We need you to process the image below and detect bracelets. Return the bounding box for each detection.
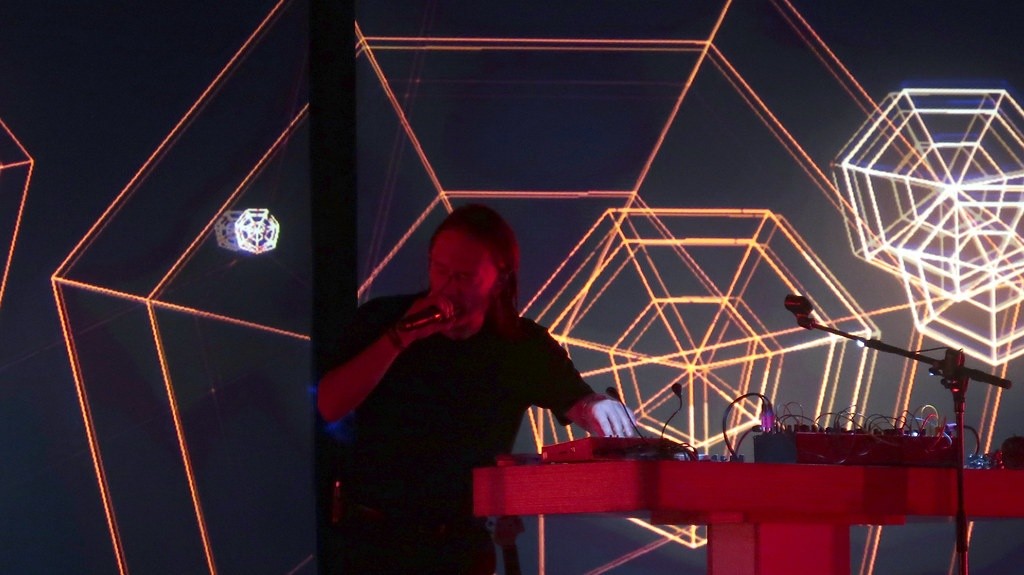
[387,326,407,354]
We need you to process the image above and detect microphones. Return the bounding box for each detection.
[660,383,683,439]
[606,387,644,439]
[397,306,442,333]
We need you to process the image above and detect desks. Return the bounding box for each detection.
[473,460,1024,575]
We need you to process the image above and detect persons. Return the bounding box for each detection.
[314,204,635,575]
